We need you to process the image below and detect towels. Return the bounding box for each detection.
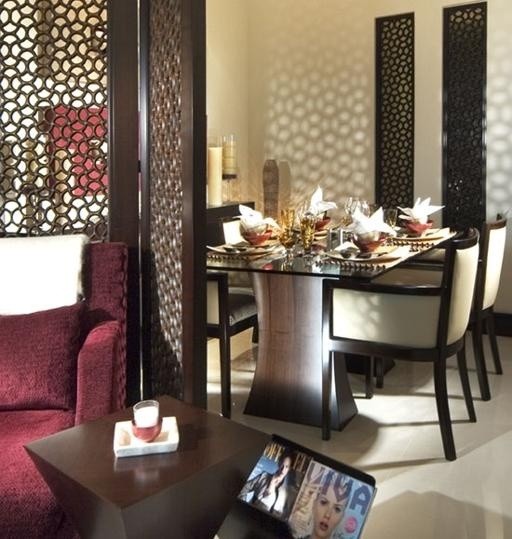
[308,185,338,219]
[336,205,399,237]
[232,203,282,232]
[396,196,446,225]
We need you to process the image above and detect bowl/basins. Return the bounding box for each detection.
[241,230,272,245]
[351,236,386,251]
[401,219,433,238]
[316,217,331,230]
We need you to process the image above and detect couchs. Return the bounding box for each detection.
[0,234,129,539]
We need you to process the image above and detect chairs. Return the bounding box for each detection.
[322,228,480,460]
[205,215,259,418]
[374,212,508,401]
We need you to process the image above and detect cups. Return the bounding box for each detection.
[132,416,162,443]
[133,399,159,427]
[344,196,397,229]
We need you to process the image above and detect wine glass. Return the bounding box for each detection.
[278,232,299,270]
[300,219,316,258]
[279,208,296,235]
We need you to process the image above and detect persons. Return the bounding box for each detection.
[237,448,298,517]
[299,469,354,538]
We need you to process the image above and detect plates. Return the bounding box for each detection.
[325,244,411,261]
[205,239,279,253]
[392,225,451,241]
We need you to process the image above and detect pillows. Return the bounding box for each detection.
[0,299,84,411]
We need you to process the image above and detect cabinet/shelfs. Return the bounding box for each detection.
[206,200,257,255]
